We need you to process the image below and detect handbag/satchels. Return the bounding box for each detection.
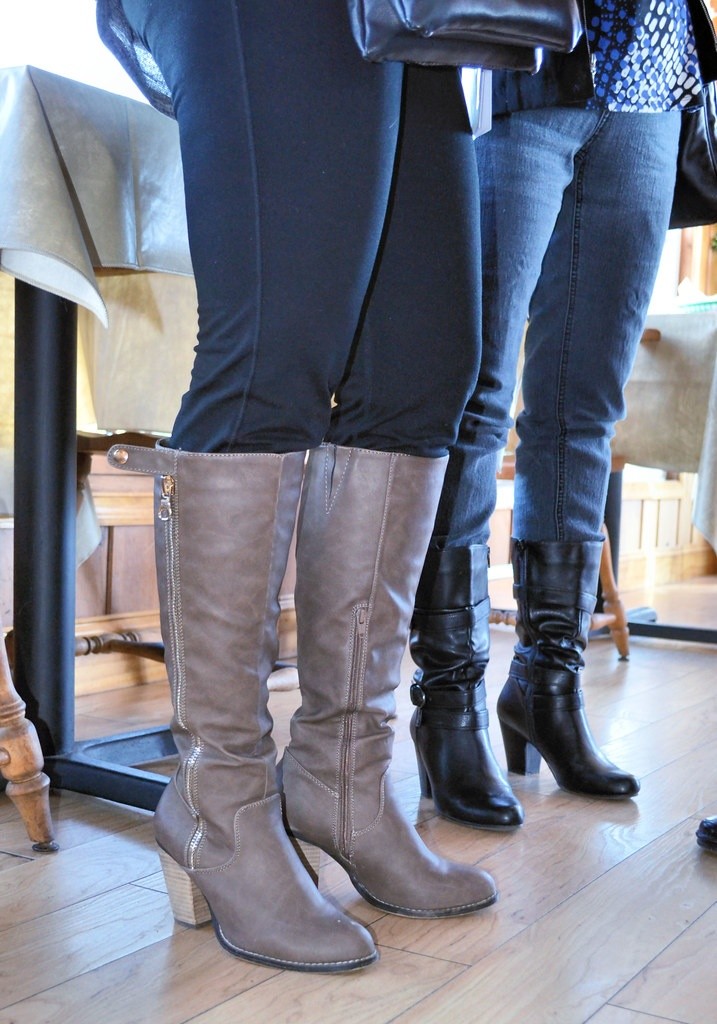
[348,0,584,74]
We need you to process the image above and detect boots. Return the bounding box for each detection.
[108,439,378,970]
[420,545,524,831]
[498,537,639,800]
[279,443,499,919]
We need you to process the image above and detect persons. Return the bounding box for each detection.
[96,0,500,973]
[408,0,717,833]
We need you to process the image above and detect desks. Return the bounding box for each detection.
[0,63,198,812]
[490,309,716,645]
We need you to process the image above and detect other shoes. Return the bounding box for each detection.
[696,815,717,852]
